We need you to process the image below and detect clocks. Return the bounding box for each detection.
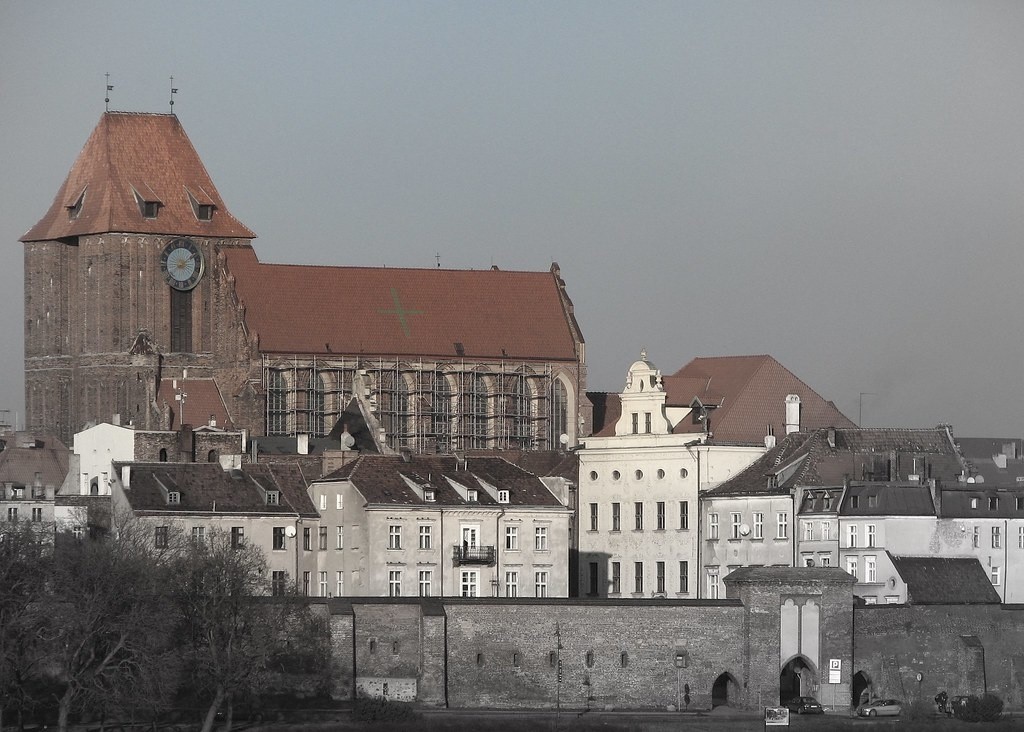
[158,236,205,292]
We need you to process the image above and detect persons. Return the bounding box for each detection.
[946,700,965,720]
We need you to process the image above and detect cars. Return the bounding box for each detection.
[785,696,822,714]
[856,698,906,718]
